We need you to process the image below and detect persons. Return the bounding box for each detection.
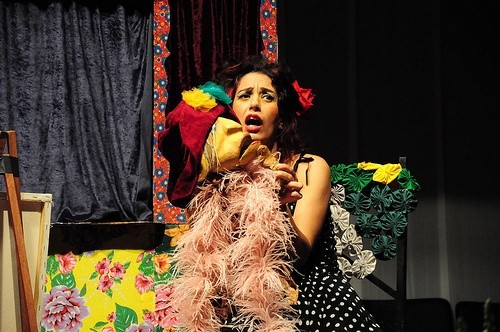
[228,58,385,332]
